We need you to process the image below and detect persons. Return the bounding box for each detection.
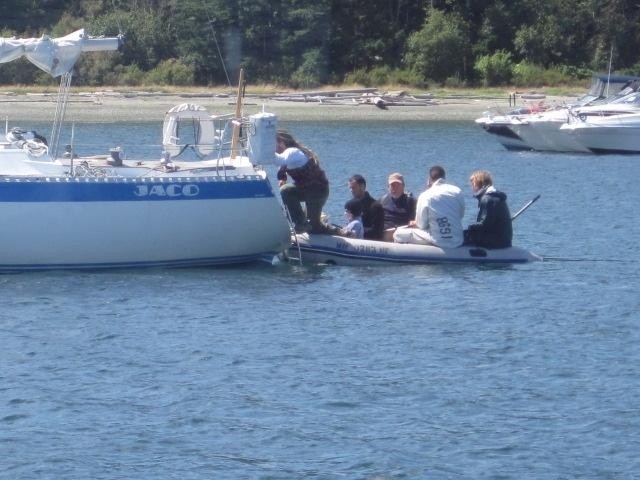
[376,170,418,241]
[273,128,330,234]
[348,174,385,241]
[391,165,466,250]
[464,169,512,248]
[338,198,366,240]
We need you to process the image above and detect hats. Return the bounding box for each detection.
[388,172,404,184]
[344,199,361,217]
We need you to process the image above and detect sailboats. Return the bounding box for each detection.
[1,29,291,274]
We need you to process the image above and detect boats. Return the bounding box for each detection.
[476,110,529,153]
[512,107,593,155]
[562,93,639,152]
[293,233,542,266]
